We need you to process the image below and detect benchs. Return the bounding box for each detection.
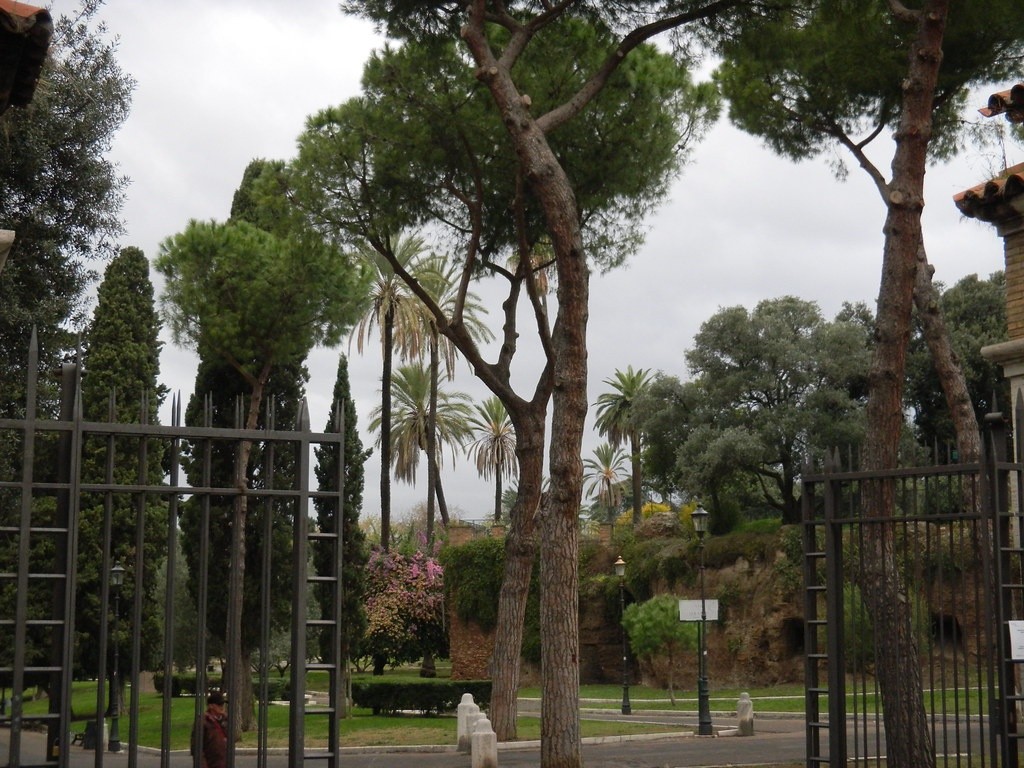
[71,720,98,746]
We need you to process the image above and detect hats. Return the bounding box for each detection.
[207,694,229,704]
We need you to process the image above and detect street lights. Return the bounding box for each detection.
[689,501,716,739]
[614,555,632,716]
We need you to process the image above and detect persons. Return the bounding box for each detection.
[190,693,228,768]
